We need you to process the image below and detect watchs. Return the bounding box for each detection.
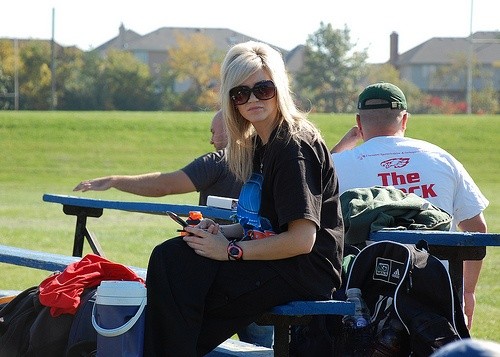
[227,239,242,261]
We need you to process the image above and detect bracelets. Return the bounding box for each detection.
[219,226,226,236]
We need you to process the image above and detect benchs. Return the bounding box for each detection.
[0,194,500,357]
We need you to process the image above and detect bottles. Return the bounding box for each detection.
[342,288,369,328]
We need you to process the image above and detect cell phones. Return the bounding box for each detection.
[166,211,224,237]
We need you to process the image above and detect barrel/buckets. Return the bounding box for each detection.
[90,281,147,357]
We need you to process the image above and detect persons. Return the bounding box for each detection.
[330,81,489,332]
[73,109,274,347]
[144,40,345,357]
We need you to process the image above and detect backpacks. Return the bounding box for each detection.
[333,240,470,357]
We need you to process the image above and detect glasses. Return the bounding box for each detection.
[229,80,277,105]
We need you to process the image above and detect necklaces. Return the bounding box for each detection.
[258,144,266,174]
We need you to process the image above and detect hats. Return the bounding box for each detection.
[358,81,407,110]
